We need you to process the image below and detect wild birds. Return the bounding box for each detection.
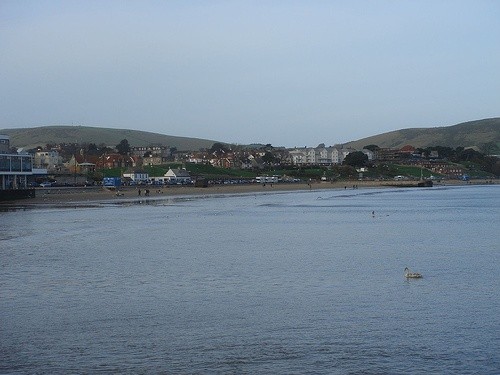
[404,266,423,278]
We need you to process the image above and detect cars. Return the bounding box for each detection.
[27,179,40,188]
[40,181,51,188]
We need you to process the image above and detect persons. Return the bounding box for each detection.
[144,189,150,197]
[262,182,358,190]
[138,188,142,197]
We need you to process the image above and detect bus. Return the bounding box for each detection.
[255,176,278,184]
